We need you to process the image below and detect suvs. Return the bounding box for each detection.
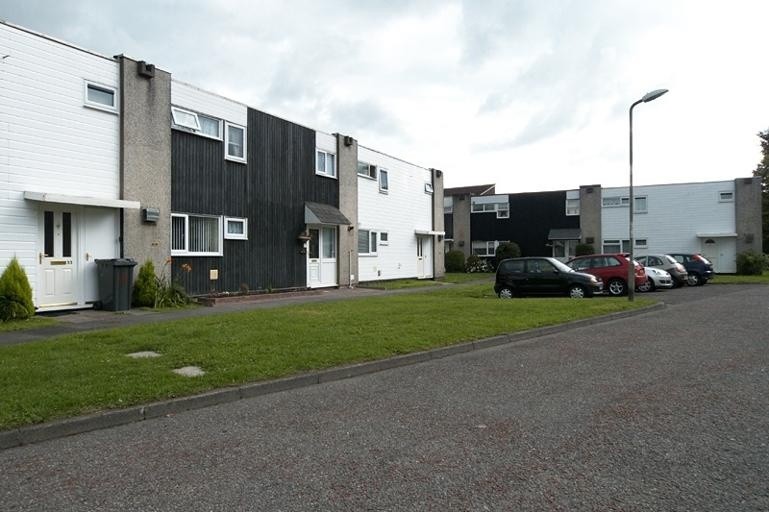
[566,252,646,296]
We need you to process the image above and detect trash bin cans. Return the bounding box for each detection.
[94,259,137,311]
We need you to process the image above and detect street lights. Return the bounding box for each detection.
[628,89,669,301]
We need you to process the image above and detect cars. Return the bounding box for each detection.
[638,265,672,291]
[494,256,602,299]
[667,253,713,285]
[634,253,688,288]
